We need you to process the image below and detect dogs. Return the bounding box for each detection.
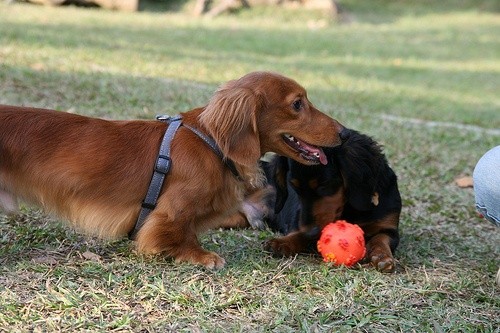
[260,128,405,274]
[0,71,351,269]
